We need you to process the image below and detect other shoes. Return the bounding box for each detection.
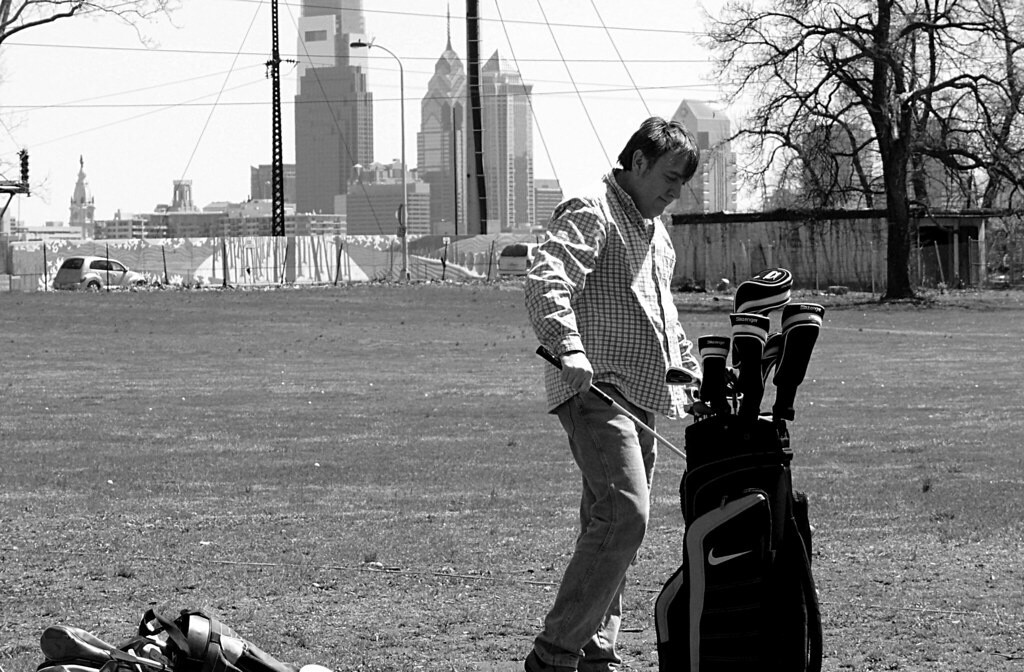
[525,648,577,672]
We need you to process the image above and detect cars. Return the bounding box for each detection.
[52,255,144,291]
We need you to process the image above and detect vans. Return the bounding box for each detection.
[498,242,541,276]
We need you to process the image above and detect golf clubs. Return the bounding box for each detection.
[664,365,745,425]
[535,344,687,460]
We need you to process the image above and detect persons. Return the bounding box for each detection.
[524,117,710,672]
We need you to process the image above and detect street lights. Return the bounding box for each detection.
[351,39,408,282]
